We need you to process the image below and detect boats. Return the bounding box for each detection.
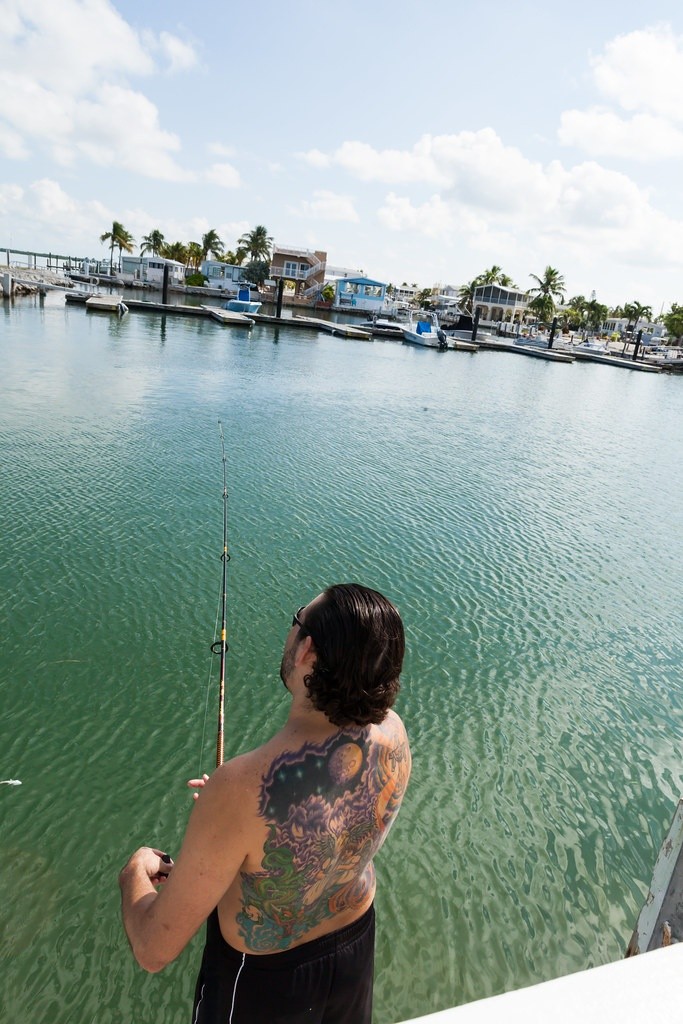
[401,310,455,348]
[513,338,548,348]
[223,283,262,313]
[450,330,491,341]
[571,343,609,356]
[642,346,683,370]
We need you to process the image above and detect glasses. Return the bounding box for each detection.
[293,606,307,632]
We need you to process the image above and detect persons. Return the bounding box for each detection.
[118,583,412,1024]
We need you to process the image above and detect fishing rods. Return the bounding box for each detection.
[209,417,232,774]
